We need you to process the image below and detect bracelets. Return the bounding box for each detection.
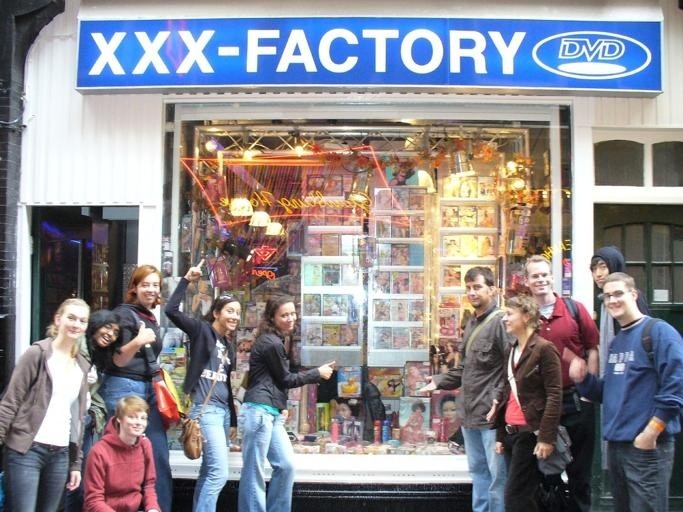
[649,420,664,433]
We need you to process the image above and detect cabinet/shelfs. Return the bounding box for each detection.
[299,174,500,369]
[37,239,109,318]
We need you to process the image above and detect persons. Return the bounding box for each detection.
[0,265,182,511]
[590,244,651,471]
[522,254,599,511]
[569,272,683,512]
[237,294,335,511]
[315,344,466,447]
[495,293,563,512]
[164,259,242,512]
[236,334,257,353]
[418,266,518,512]
[160,355,176,375]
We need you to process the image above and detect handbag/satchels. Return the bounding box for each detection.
[538,424,573,475]
[152,368,187,432]
[178,418,202,460]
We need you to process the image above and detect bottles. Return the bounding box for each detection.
[382,420,392,445]
[373,420,381,444]
[332,419,339,443]
[438,417,449,442]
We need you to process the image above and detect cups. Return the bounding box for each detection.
[392,429,400,441]
